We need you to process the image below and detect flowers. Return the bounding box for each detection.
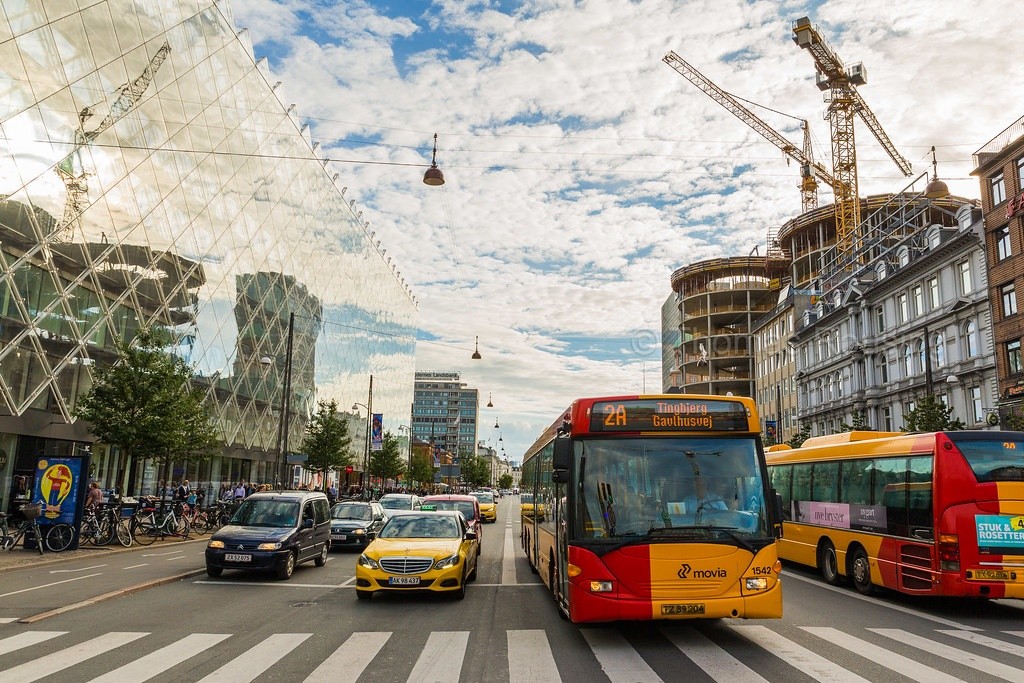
[1009,383,1024,395]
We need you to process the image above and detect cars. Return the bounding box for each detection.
[520,493,544,521]
[356,505,478,601]
[468,492,498,523]
[379,494,482,555]
[499,490,513,495]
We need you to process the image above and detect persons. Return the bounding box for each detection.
[299,483,336,502]
[338,480,464,500]
[6,476,26,528]
[220,480,270,517]
[682,475,728,513]
[85,483,103,517]
[158,478,198,516]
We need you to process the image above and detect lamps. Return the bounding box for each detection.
[946,371,966,386]
[487,392,493,408]
[494,417,507,457]
[423,133,445,186]
[791,416,800,420]
[471,335,482,360]
[925,146,949,202]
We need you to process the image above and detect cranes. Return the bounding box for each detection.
[57,42,172,246]
[662,50,852,216]
[792,16,914,272]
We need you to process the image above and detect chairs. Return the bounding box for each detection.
[886,506,930,536]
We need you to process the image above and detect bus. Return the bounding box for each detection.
[521,394,785,624]
[737,430,1024,599]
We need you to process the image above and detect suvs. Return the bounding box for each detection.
[330,500,389,551]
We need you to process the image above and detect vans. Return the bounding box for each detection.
[207,490,331,580]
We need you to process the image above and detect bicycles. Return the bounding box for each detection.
[0,500,45,554]
[128,500,195,546]
[194,497,244,535]
[41,508,77,552]
[79,505,133,547]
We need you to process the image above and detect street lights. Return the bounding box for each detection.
[398,403,412,491]
[776,385,800,444]
[923,325,959,409]
[260,312,294,490]
[352,374,372,490]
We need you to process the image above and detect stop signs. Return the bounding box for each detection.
[346,465,353,474]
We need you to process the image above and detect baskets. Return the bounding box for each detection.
[19,504,41,519]
